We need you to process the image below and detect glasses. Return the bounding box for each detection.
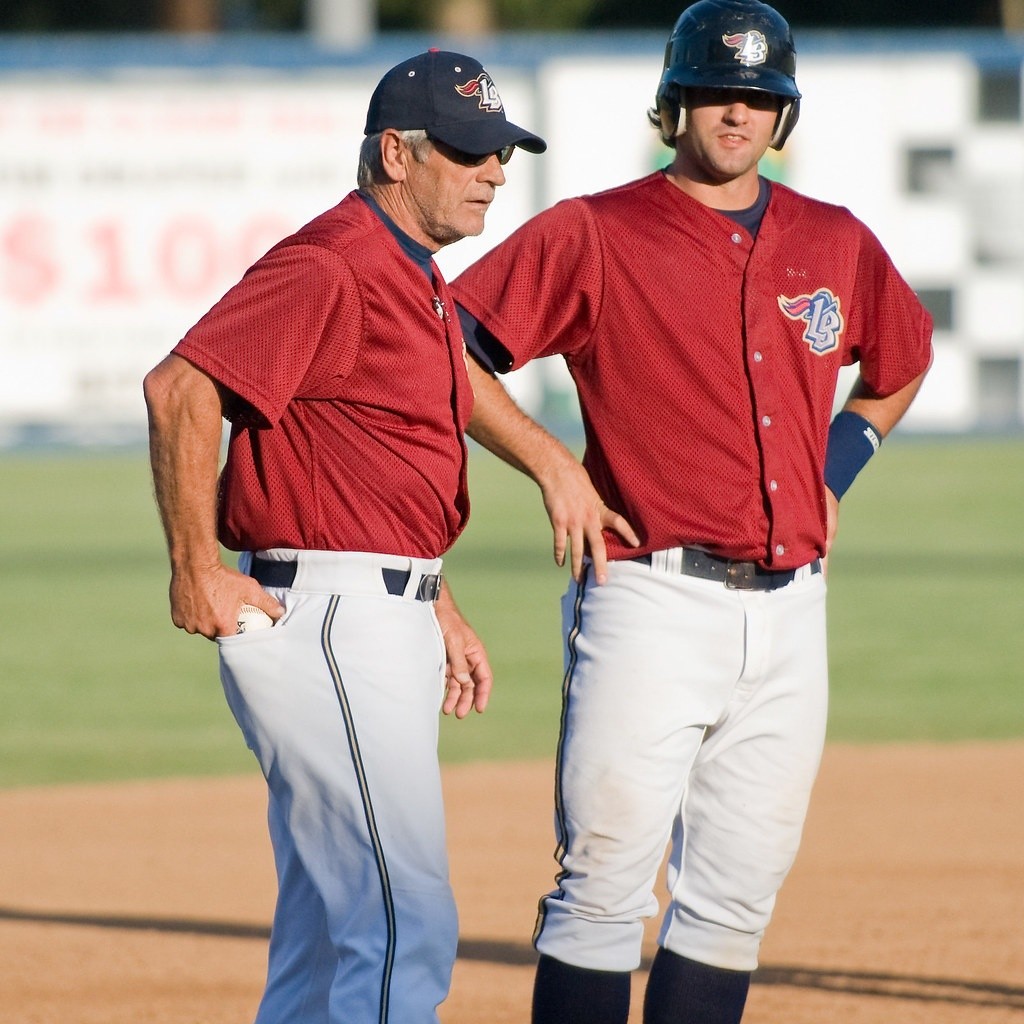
[427,135,515,167]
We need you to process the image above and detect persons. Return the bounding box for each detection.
[443,1,936,1023]
[142,45,548,1023]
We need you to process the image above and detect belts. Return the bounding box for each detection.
[629,546,821,591]
[250,554,442,604]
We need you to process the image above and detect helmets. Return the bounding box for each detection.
[656,0,802,151]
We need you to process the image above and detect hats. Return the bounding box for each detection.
[364,49,547,154]
